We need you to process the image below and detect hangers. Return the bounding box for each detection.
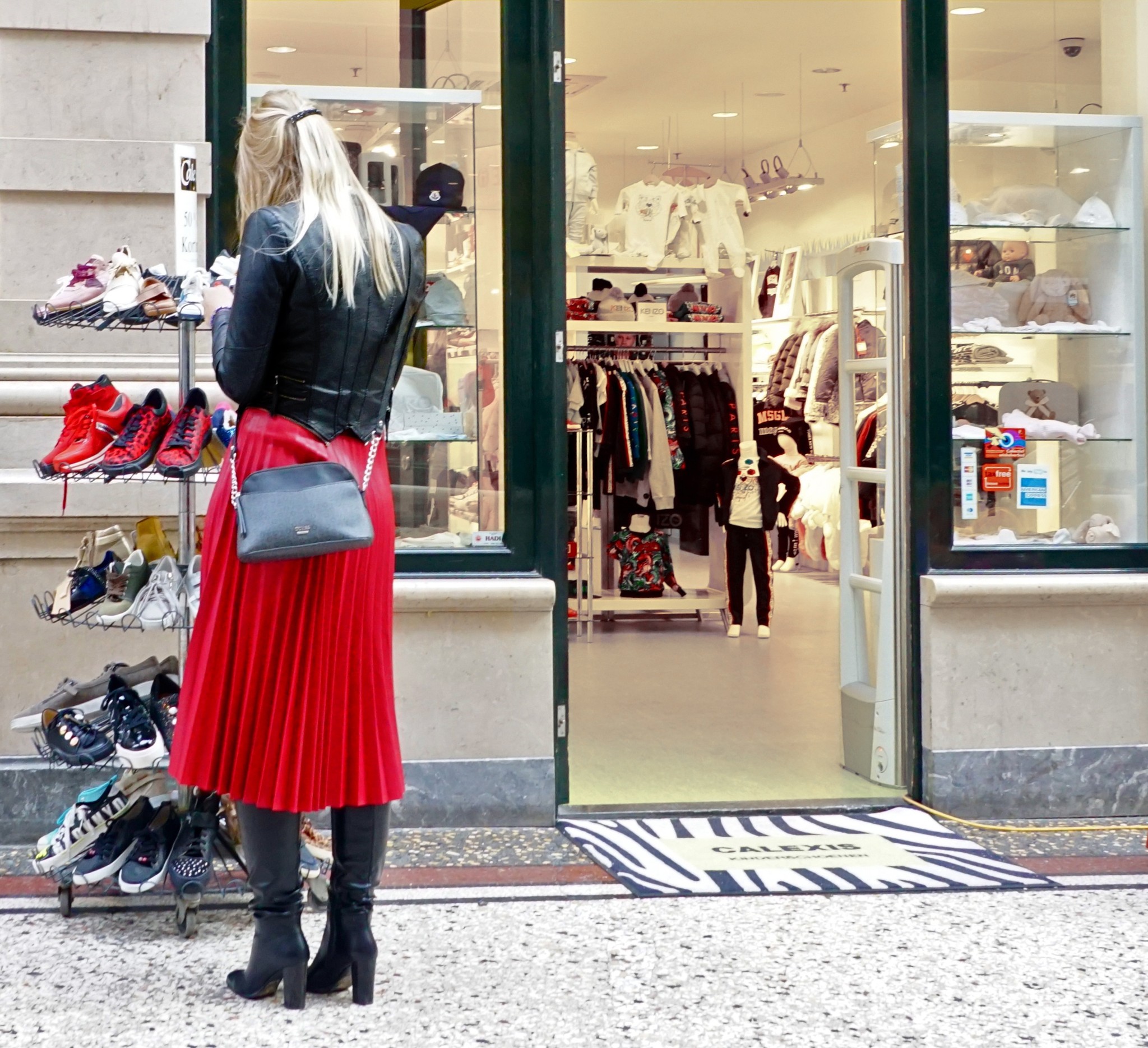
[644,161,716,188]
[566,345,723,375]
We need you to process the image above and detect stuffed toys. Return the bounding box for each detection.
[1072,513,1121,543]
[1026,389,1056,420]
[1017,269,1092,326]
[579,227,622,255]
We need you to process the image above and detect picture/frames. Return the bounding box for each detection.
[772,247,802,318]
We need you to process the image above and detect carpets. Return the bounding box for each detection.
[557,805,1062,896]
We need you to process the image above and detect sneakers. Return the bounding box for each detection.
[10,656,180,766]
[45,244,241,318]
[50,516,202,628]
[39,373,237,478]
[32,771,333,895]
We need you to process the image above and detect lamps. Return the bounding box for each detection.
[721,54,823,202]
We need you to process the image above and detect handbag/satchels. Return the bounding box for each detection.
[236,462,374,564]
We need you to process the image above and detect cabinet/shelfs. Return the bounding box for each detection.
[565,254,756,613]
[31,273,332,930]
[867,109,1148,551]
[246,83,484,549]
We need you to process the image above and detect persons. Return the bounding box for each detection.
[603,333,643,360]
[565,129,598,246]
[449,466,479,505]
[713,441,801,638]
[166,88,425,1011]
[606,514,686,598]
[771,420,810,572]
[974,241,1034,282]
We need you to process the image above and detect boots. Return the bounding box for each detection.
[306,885,378,1006]
[226,903,310,1010]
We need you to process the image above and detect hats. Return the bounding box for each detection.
[586,278,699,321]
[1071,191,1116,227]
[458,364,495,408]
[415,163,465,208]
[413,278,470,328]
